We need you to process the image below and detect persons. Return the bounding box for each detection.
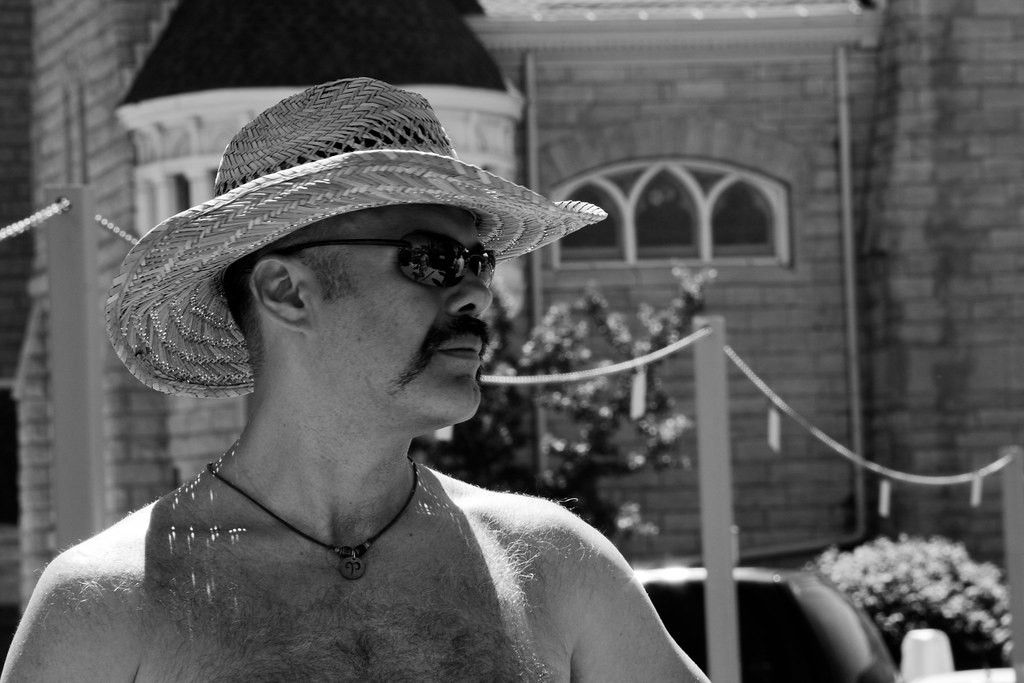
[0,79,712,683]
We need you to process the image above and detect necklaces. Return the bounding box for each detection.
[206,454,417,579]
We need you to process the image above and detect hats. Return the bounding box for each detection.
[102,77,605,397]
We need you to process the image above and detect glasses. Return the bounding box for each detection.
[270,229,497,299]
